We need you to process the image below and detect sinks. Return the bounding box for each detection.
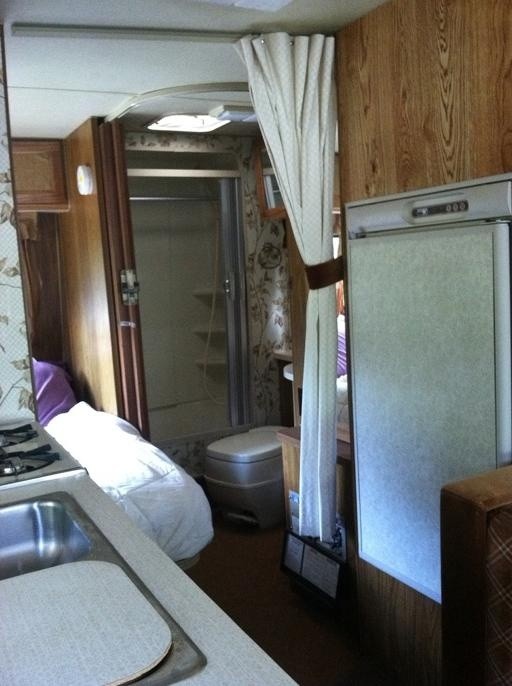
[0,561,207,685]
[0,491,98,581]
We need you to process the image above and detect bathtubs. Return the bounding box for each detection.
[148,397,255,479]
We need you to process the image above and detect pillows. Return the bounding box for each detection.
[33,359,78,429]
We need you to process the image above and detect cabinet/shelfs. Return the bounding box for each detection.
[276,423,355,614]
[11,140,68,213]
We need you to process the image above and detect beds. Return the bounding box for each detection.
[33,361,200,570]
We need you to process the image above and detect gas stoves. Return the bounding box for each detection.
[0,419,84,486]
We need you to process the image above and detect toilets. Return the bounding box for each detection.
[205,423,291,529]
